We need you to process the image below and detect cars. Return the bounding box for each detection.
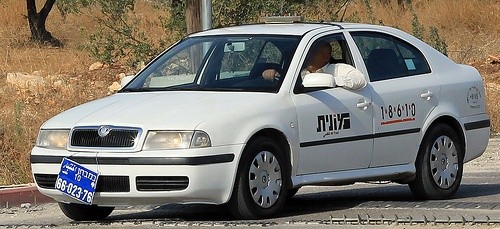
[29,16,490,220]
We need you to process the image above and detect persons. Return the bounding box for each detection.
[262,41,367,91]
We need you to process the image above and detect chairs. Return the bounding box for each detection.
[249,62,281,78]
[366,49,401,82]
